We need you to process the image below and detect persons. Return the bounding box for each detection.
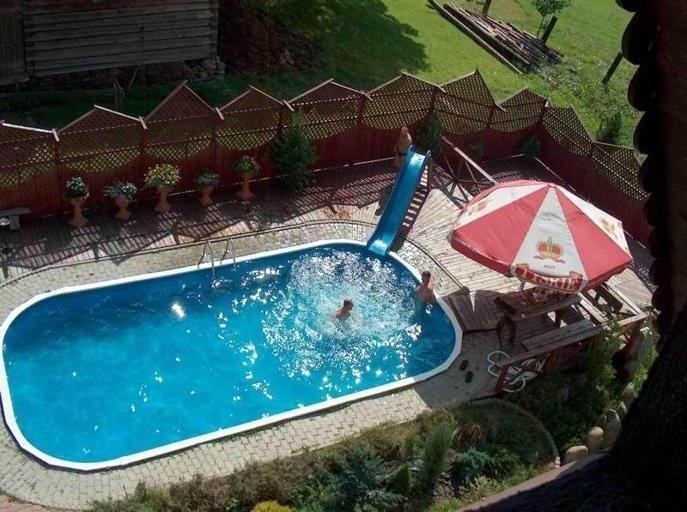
[415,270,433,304]
[393,126,412,169]
[333,298,354,321]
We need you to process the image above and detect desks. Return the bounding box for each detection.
[494,286,581,346]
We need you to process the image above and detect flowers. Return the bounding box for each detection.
[234,154,259,176]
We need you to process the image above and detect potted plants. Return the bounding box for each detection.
[60,161,219,228]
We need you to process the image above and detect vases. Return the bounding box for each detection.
[235,172,255,200]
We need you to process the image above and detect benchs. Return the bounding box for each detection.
[520,319,593,351]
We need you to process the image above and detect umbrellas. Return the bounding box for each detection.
[446,178,635,294]
